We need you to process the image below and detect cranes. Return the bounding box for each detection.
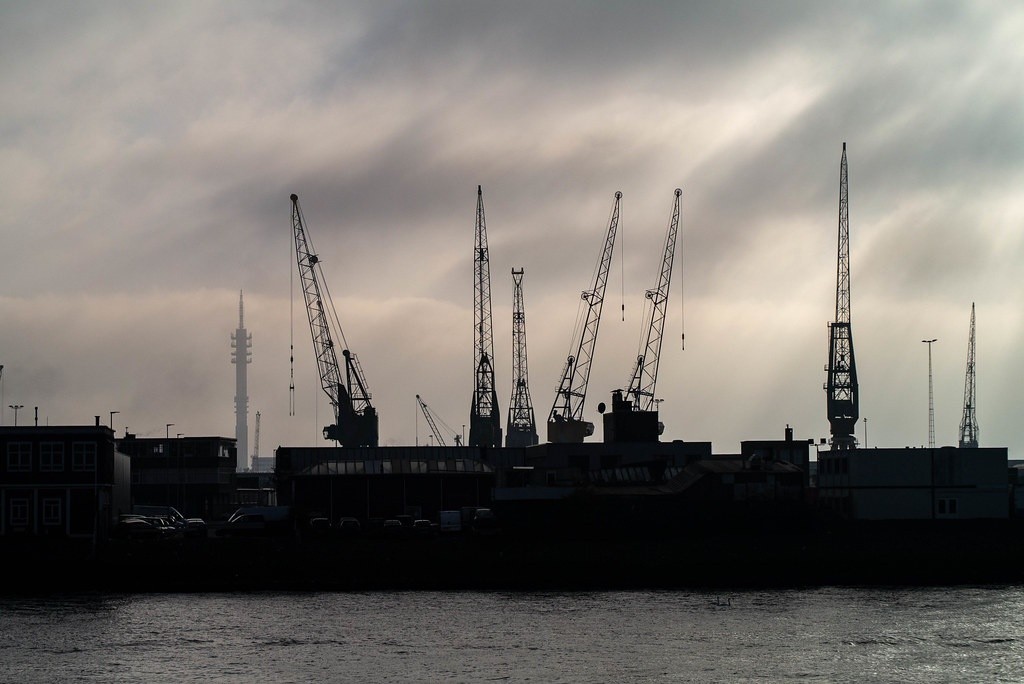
[958,301,980,446]
[822,141,861,448]
[503,267,539,447]
[252,411,262,471]
[547,191,624,442]
[468,185,503,448]
[290,193,381,449]
[597,187,686,442]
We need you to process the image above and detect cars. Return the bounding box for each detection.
[310,518,332,528]
[112,504,207,541]
[412,520,432,528]
[384,520,402,527]
[340,520,361,528]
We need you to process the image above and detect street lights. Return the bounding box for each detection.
[177,433,184,438]
[166,423,175,437]
[110,411,120,429]
[922,338,938,448]
[9,405,24,426]
